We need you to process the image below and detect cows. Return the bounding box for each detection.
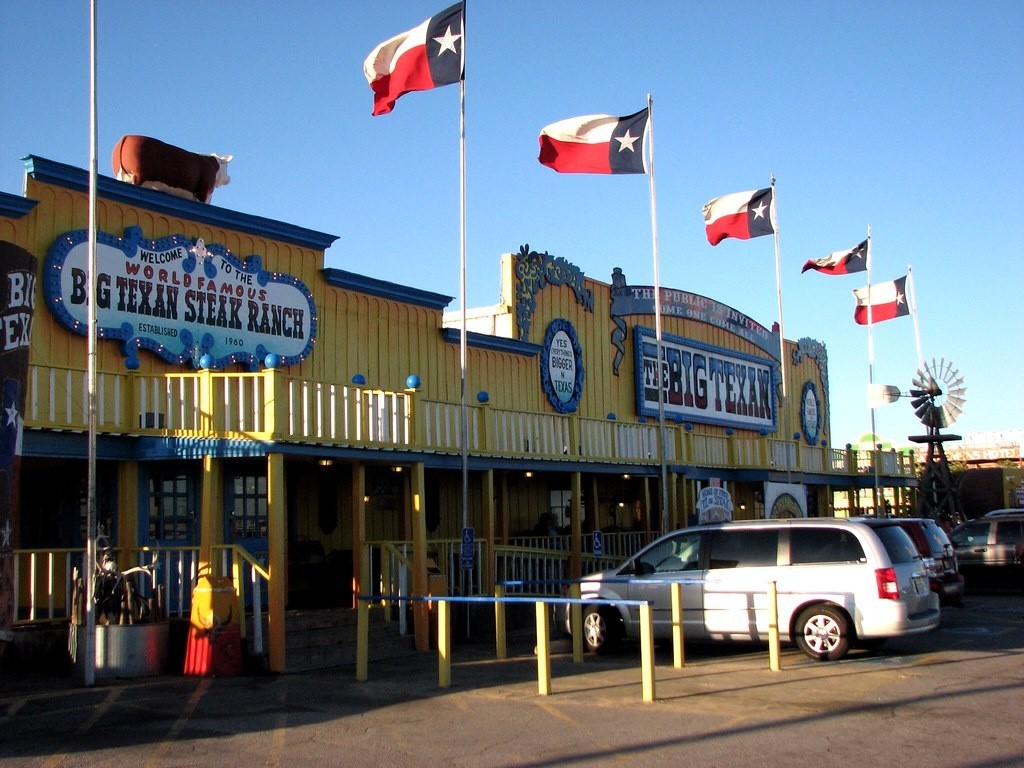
[112,134,234,206]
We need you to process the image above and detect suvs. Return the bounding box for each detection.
[858,510,966,596]
[553,508,941,663]
[939,510,1024,585]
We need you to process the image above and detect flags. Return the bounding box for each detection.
[853,273,912,325]
[801,239,867,275]
[364,0,466,116]
[537,107,650,175]
[703,187,775,246]
